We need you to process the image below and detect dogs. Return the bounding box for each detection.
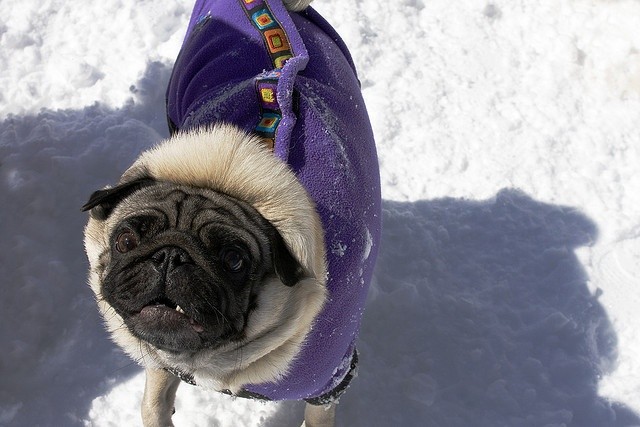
[79,0,383,426]
